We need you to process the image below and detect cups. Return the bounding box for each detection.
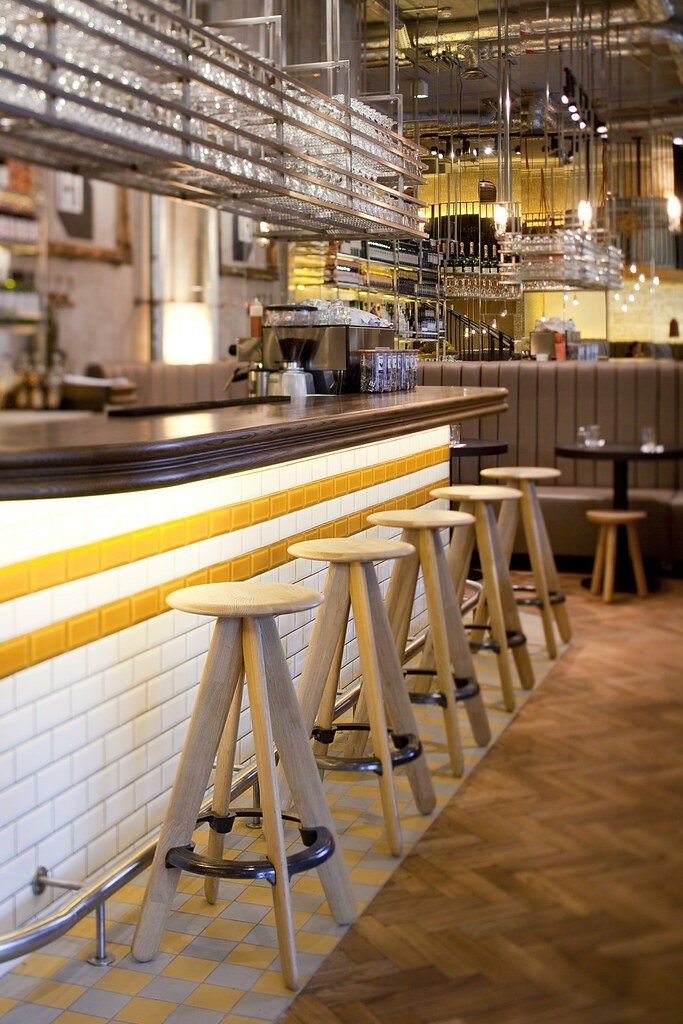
[641,428,655,450]
[449,425,460,445]
[585,425,600,446]
[265,299,352,325]
[536,354,548,361]
[502,231,624,290]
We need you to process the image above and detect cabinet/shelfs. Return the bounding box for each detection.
[285,233,447,354]
[1,162,50,377]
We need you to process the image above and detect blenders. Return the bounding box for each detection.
[265,305,318,394]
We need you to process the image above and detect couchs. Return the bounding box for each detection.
[413,362,683,559]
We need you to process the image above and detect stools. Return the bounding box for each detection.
[129,466,649,992]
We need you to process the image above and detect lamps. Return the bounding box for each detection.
[432,67,613,165]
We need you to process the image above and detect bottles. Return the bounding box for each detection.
[368,242,394,290]
[578,427,586,447]
[250,297,264,336]
[447,276,523,298]
[447,242,521,273]
[567,319,574,341]
[418,304,444,338]
[337,257,367,291]
[396,238,446,298]
[397,303,414,338]
[364,302,394,323]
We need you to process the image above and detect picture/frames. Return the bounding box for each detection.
[45,166,134,266]
[218,212,280,280]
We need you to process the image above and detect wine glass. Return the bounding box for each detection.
[0,0,421,233]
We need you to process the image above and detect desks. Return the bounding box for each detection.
[450,440,509,580]
[555,442,683,595]
